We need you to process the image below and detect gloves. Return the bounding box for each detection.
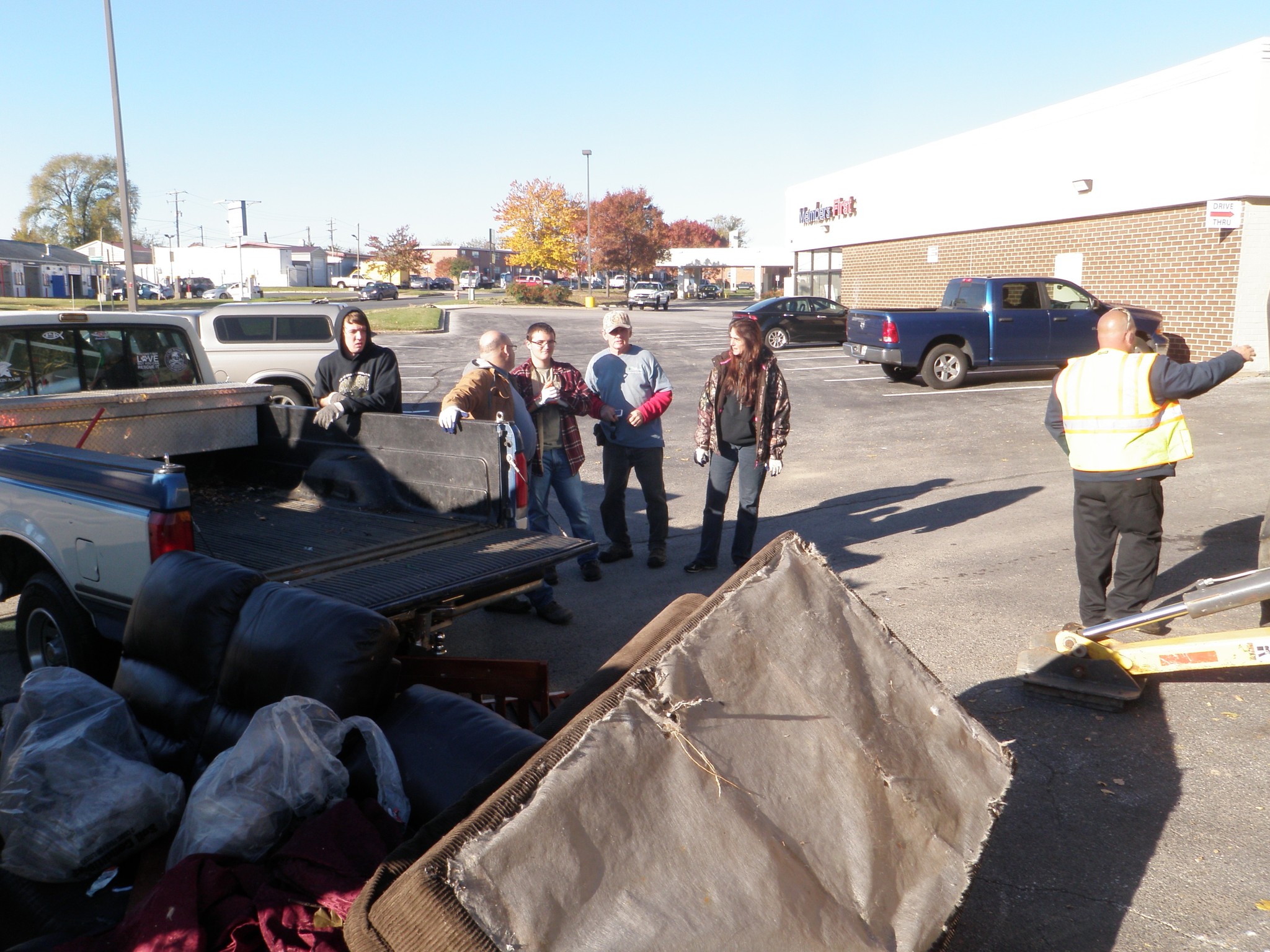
[535,380,569,408]
[694,445,710,467]
[326,392,347,404]
[764,457,783,477]
[438,406,469,434]
[313,402,345,430]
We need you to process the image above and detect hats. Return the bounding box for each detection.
[602,310,632,334]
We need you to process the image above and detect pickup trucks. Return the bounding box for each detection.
[331,274,383,289]
[1,308,595,689]
[609,275,634,288]
[629,281,670,311]
[125,300,347,409]
[736,280,755,290]
[846,276,1169,388]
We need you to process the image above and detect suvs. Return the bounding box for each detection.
[171,277,214,297]
[515,275,553,288]
[698,278,710,288]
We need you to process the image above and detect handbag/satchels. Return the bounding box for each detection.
[593,424,606,447]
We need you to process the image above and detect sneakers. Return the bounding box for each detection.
[1130,619,1161,635]
[536,600,575,624]
[490,597,531,615]
[647,547,667,567]
[684,560,745,573]
[598,545,633,563]
[544,566,559,585]
[580,560,602,582]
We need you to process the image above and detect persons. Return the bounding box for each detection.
[1044,306,1256,639]
[510,322,604,583]
[438,329,574,624]
[685,317,791,574]
[313,305,405,430]
[583,309,670,569]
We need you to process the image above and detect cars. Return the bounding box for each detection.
[663,281,679,299]
[430,278,454,290]
[698,284,721,300]
[114,280,172,302]
[591,282,603,289]
[556,280,570,289]
[408,274,420,281]
[203,282,260,302]
[570,282,579,290]
[358,282,400,301]
[734,293,849,350]
[412,276,431,290]
[578,281,591,288]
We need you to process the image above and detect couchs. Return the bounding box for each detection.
[345,529,1022,950]
[103,553,389,813]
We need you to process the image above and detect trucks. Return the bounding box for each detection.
[584,276,602,284]
[348,263,408,289]
[459,270,493,291]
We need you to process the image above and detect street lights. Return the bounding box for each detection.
[353,222,362,279]
[583,149,596,276]
[164,232,176,284]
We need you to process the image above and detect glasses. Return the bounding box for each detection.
[501,345,517,351]
[1109,306,1131,332]
[531,340,557,347]
